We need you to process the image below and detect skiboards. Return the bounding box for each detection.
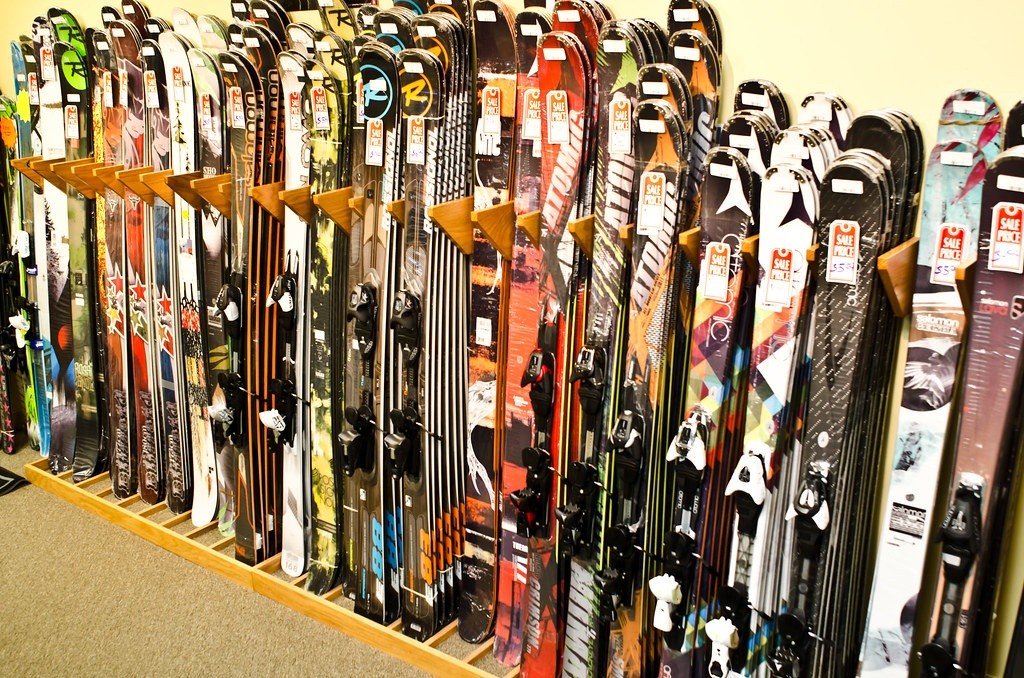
[0,0,1023,678]
